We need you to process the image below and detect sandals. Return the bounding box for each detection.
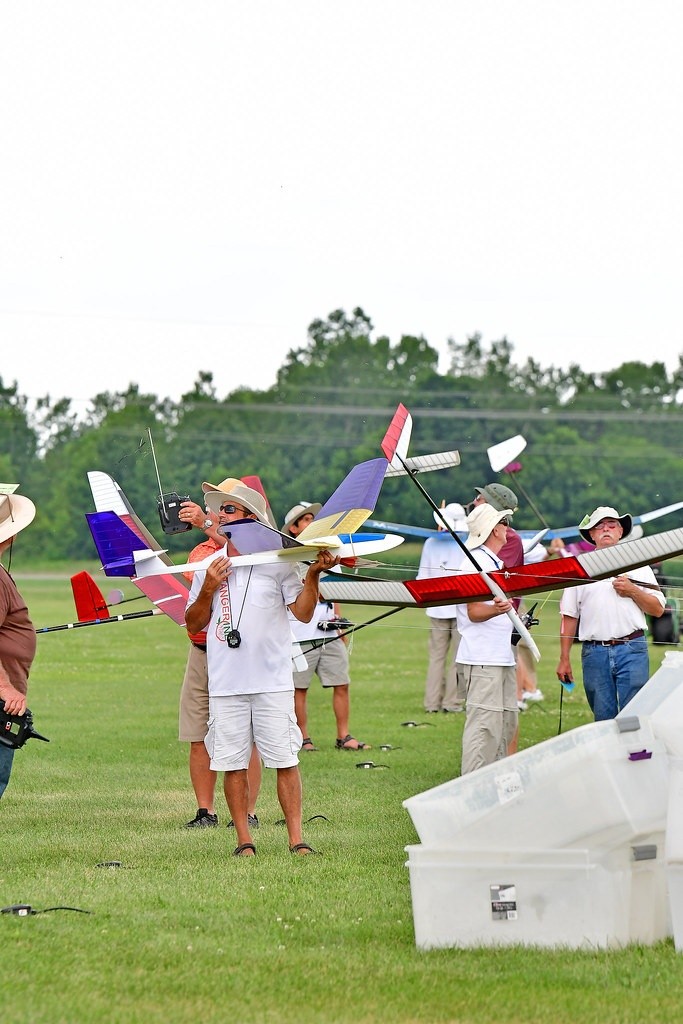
[336,734,373,750]
[289,842,324,857]
[303,738,320,752]
[233,843,257,858]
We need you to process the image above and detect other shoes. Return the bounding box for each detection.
[426,707,463,714]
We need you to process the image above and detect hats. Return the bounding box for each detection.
[475,483,518,512]
[433,503,470,531]
[578,507,633,546]
[201,478,247,493]
[0,494,36,542]
[204,486,273,534]
[281,502,322,536]
[464,503,515,551]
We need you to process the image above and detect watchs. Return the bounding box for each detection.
[202,519,214,531]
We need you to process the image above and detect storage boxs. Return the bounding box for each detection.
[402,650,683,956]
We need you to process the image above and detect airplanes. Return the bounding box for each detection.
[35,400,683,664]
[85,459,407,584]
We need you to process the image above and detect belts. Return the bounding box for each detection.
[583,630,646,647]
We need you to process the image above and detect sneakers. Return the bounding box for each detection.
[518,690,544,710]
[181,808,218,828]
[226,812,259,829]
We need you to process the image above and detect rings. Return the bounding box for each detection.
[325,561,330,564]
[187,513,191,517]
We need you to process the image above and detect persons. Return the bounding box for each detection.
[185,485,342,856]
[556,508,666,724]
[471,482,565,754]
[0,482,37,795]
[415,502,466,713]
[280,503,375,751]
[455,504,519,779]
[178,478,263,826]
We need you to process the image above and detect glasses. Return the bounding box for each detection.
[594,520,622,530]
[219,505,252,515]
[498,517,509,526]
